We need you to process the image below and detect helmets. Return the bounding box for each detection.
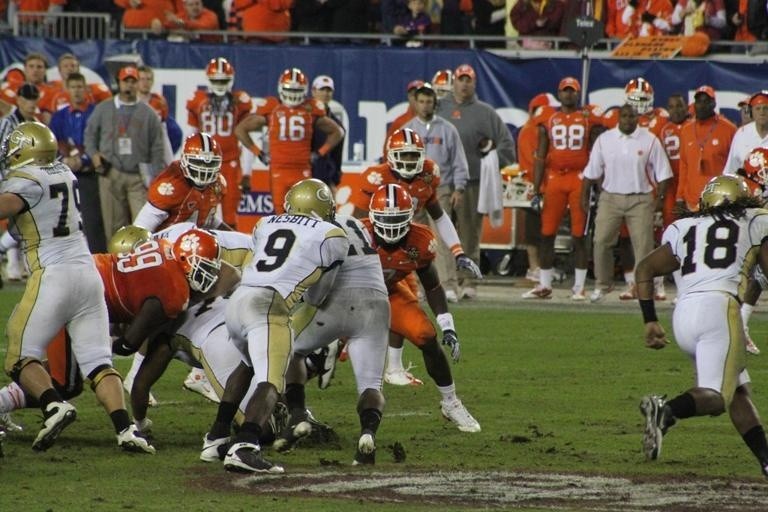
[368,183,414,245]
[283,178,336,223]
[171,230,220,294]
[179,132,222,190]
[743,148,767,187]
[624,77,653,114]
[206,57,234,96]
[431,70,455,100]
[276,67,308,107]
[699,174,751,211]
[106,225,153,254]
[0,121,57,178]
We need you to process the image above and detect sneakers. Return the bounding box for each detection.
[742,328,759,356]
[522,285,551,299]
[182,371,220,404]
[123,374,157,407]
[199,432,236,463]
[382,362,423,387]
[32,401,76,451]
[439,398,481,433]
[351,429,376,465]
[444,289,457,303]
[571,288,585,301]
[461,287,475,298]
[271,415,312,454]
[638,395,674,462]
[116,425,155,455]
[312,339,344,389]
[340,340,350,362]
[0,412,22,433]
[618,280,638,299]
[590,283,614,304]
[223,442,284,475]
[525,266,554,282]
[653,281,666,300]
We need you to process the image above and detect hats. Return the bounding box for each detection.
[17,84,38,99]
[118,65,139,80]
[528,92,561,108]
[748,92,767,105]
[406,80,423,90]
[559,76,579,91]
[693,85,714,98]
[312,74,333,91]
[737,99,750,106]
[454,64,475,79]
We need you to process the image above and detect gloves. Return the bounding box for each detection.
[530,194,540,213]
[441,328,460,363]
[455,255,482,280]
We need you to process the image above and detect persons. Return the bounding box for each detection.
[128,130,231,234]
[83,65,166,237]
[107,224,255,406]
[4,0,67,33]
[182,54,254,228]
[432,64,518,299]
[308,72,347,208]
[719,90,767,203]
[128,68,177,170]
[674,84,740,218]
[351,182,482,436]
[389,87,473,305]
[230,1,294,43]
[159,0,223,44]
[382,79,427,162]
[522,74,602,304]
[595,78,679,302]
[578,100,674,302]
[307,338,348,392]
[629,172,766,469]
[354,125,483,389]
[396,0,767,58]
[660,89,690,230]
[0,229,223,437]
[1,83,41,135]
[273,212,393,467]
[0,53,45,92]
[199,177,348,475]
[112,1,175,37]
[129,294,288,463]
[44,50,111,112]
[516,88,570,283]
[0,120,159,457]
[737,97,754,126]
[232,65,348,218]
[45,73,102,147]
